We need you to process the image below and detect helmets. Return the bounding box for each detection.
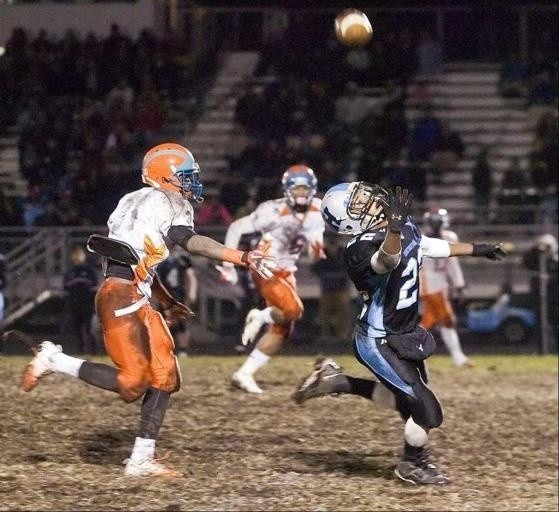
[141,142,201,203]
[281,164,318,207]
[422,209,446,231]
[322,181,390,235]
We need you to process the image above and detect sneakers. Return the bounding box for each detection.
[22,340,56,392]
[395,460,450,486]
[123,457,183,479]
[242,308,260,346]
[232,375,263,394]
[293,356,342,403]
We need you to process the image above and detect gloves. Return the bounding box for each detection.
[240,242,273,280]
[472,241,506,261]
[310,241,326,262]
[165,298,195,326]
[374,186,414,233]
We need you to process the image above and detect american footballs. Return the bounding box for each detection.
[334,7,375,48]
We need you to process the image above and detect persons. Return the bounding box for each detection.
[207,163,325,395]
[24,142,142,226]
[512,182,558,224]
[152,245,197,358]
[229,20,277,140]
[326,13,449,182]
[212,144,274,221]
[0,23,219,141]
[279,26,324,164]
[418,211,476,369]
[312,230,356,340]
[473,113,559,181]
[499,54,559,105]
[61,245,103,355]
[439,210,458,242]
[522,235,559,351]
[292,183,509,484]
[23,142,277,474]
